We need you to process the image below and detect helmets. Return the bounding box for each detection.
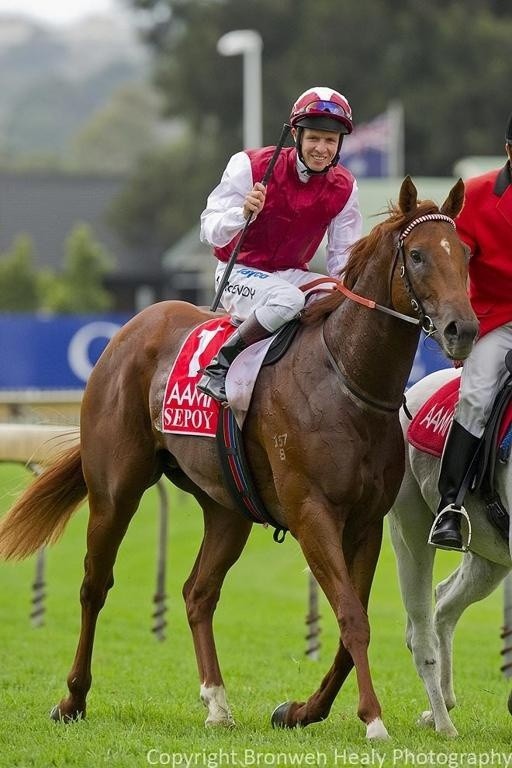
[289,87,354,134]
[505,114,512,146]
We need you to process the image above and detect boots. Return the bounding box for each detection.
[197,327,247,402]
[431,417,484,549]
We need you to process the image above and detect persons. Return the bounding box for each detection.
[194,86,366,410]
[428,115,512,545]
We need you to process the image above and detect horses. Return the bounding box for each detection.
[386,357,512,742]
[1,175,482,745]
